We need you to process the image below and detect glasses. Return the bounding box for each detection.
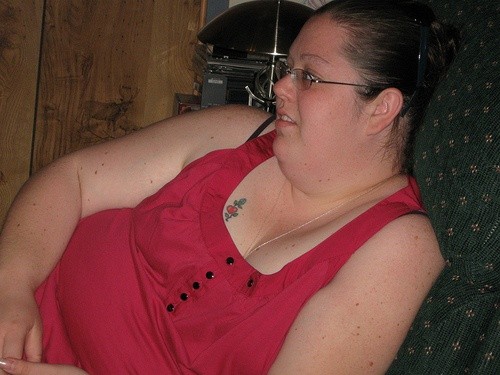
[276,60,374,90]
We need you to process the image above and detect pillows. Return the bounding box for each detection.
[381,0,500,375]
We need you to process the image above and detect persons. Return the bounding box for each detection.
[0,0,443,375]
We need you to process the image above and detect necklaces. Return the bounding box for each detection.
[246,181,381,257]
